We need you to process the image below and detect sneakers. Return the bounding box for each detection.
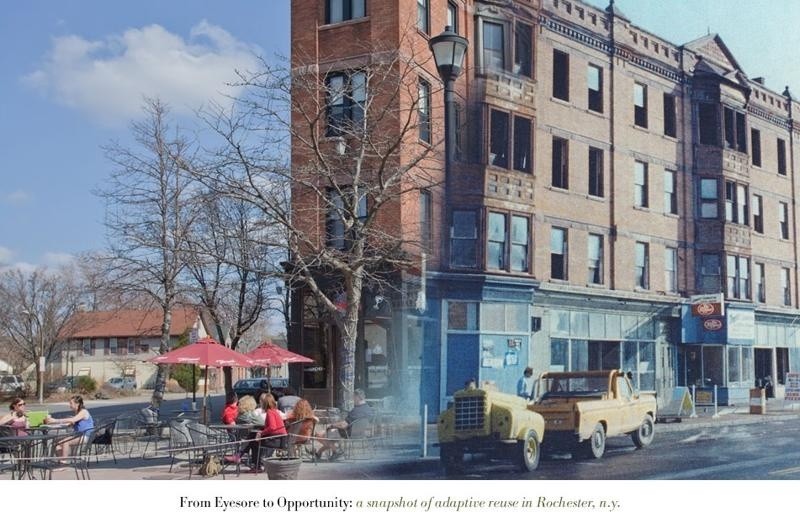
[225,453,241,464]
[248,466,265,473]
[328,448,345,460]
[306,445,321,459]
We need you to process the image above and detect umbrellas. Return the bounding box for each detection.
[245,339,315,388]
[142,335,270,426]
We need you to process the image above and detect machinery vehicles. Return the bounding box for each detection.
[436,381,546,472]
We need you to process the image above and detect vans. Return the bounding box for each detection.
[0,375,27,396]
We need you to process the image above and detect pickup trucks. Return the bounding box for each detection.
[526,369,658,461]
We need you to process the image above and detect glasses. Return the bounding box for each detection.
[17,402,25,406]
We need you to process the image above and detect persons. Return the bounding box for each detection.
[0,396,35,471]
[220,382,374,474]
[44,395,98,471]
[516,365,535,400]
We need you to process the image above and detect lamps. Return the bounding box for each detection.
[334,135,352,162]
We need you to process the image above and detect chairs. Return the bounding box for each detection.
[0,394,402,480]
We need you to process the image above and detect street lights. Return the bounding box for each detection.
[70,353,74,388]
[19,310,44,404]
[427,24,469,274]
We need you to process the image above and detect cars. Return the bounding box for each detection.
[106,377,138,392]
[233,377,297,404]
[45,376,95,396]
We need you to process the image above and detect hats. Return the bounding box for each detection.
[526,367,533,372]
